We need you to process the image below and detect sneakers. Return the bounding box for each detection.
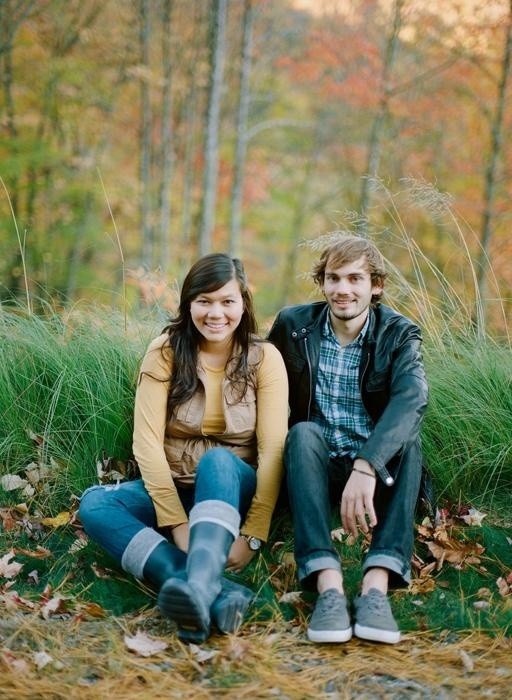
[351,587,404,646]
[304,588,353,646]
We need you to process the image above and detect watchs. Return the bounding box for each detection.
[242,535,262,552]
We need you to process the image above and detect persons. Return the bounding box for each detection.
[267,236,429,645]
[79,255,291,644]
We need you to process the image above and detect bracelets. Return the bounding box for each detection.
[351,468,377,478]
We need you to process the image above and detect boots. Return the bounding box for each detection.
[154,498,241,645]
[119,524,257,640]
